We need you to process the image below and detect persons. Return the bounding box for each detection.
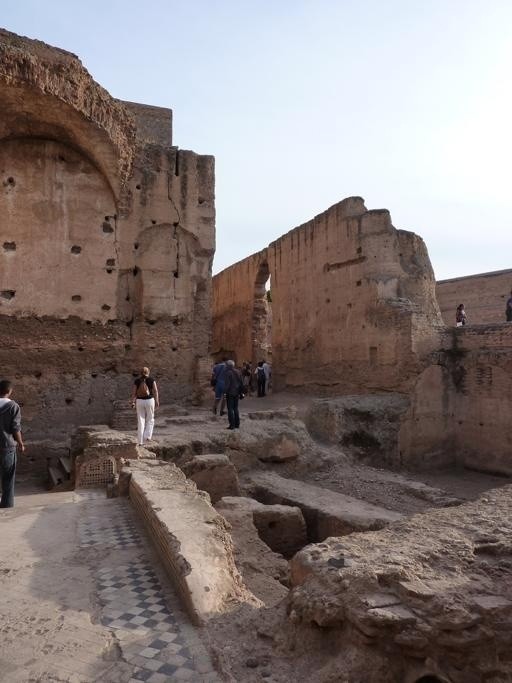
[133,366,160,445]
[210,356,269,429]
[455,302,467,323]
[1,378,26,509]
[506,299,512,321]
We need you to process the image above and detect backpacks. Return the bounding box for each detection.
[137,379,150,397]
